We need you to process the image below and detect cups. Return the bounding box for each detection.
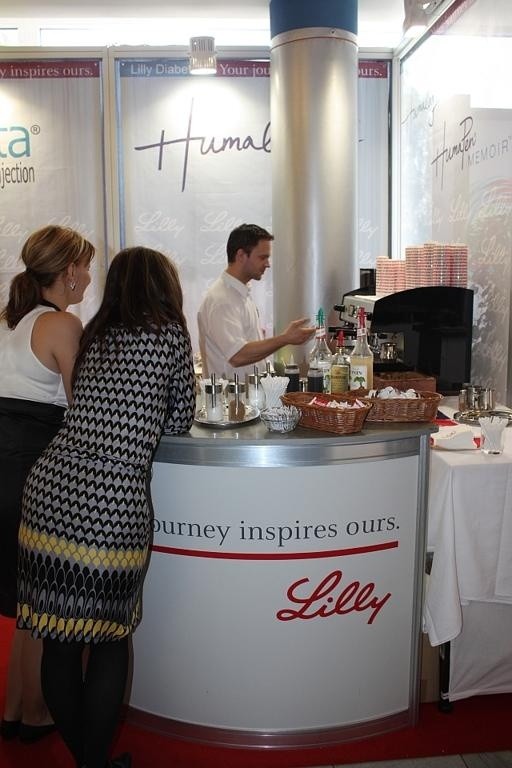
[380,341,397,363]
[467,386,496,411]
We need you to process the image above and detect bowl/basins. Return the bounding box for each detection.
[374,241,469,298]
[260,406,302,434]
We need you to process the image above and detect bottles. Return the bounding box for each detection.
[307,367,323,394]
[307,326,332,394]
[349,326,373,391]
[285,364,301,394]
[332,346,349,395]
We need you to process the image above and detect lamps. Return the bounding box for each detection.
[189,36,216,76]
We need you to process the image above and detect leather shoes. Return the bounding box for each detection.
[25,719,57,737]
[0,718,20,731]
[115,753,131,766]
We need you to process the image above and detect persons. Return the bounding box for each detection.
[198,226,319,394]
[15,248,196,767]
[3,227,94,742]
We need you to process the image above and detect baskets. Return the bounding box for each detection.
[279,392,373,434]
[345,389,444,422]
[375,371,437,393]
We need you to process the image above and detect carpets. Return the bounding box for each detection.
[0,614,512,768]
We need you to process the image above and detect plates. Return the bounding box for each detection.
[454,410,512,429]
[196,406,261,428]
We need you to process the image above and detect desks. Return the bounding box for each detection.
[424,394,512,712]
